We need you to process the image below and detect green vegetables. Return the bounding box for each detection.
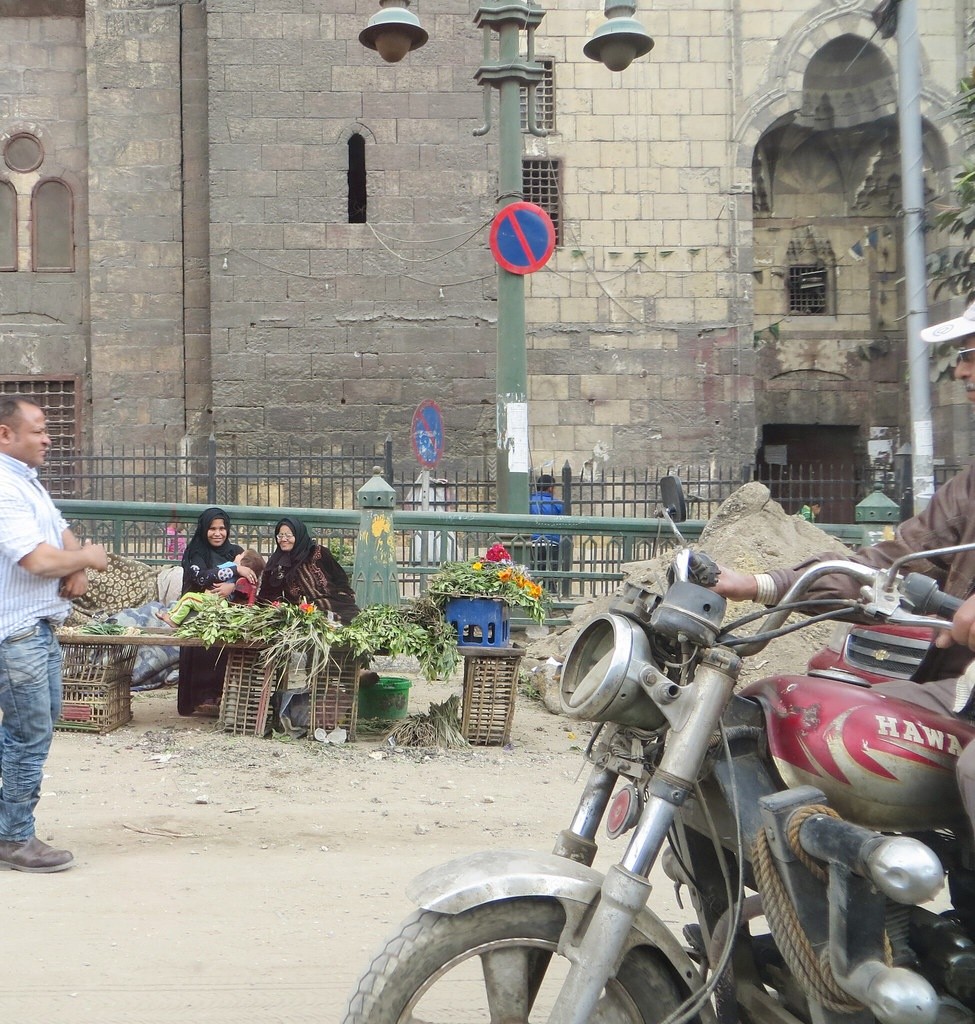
[430,557,554,626]
[173,596,461,684]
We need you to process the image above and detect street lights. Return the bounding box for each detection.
[359,1,656,631]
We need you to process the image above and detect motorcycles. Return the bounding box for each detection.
[344,476,975,1024]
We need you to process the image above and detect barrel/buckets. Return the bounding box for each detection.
[358,678,412,719]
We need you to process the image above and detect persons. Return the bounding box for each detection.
[529,475,562,594]
[0,396,108,872]
[683,302,975,971]
[156,508,380,718]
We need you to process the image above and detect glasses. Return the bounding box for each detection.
[956,346,975,362]
[276,533,294,540]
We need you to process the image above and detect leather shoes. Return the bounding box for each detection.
[0,836,81,874]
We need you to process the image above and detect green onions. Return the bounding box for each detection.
[57,623,142,637]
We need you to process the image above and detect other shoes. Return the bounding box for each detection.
[152,612,179,629]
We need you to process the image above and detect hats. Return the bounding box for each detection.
[920,301,975,343]
